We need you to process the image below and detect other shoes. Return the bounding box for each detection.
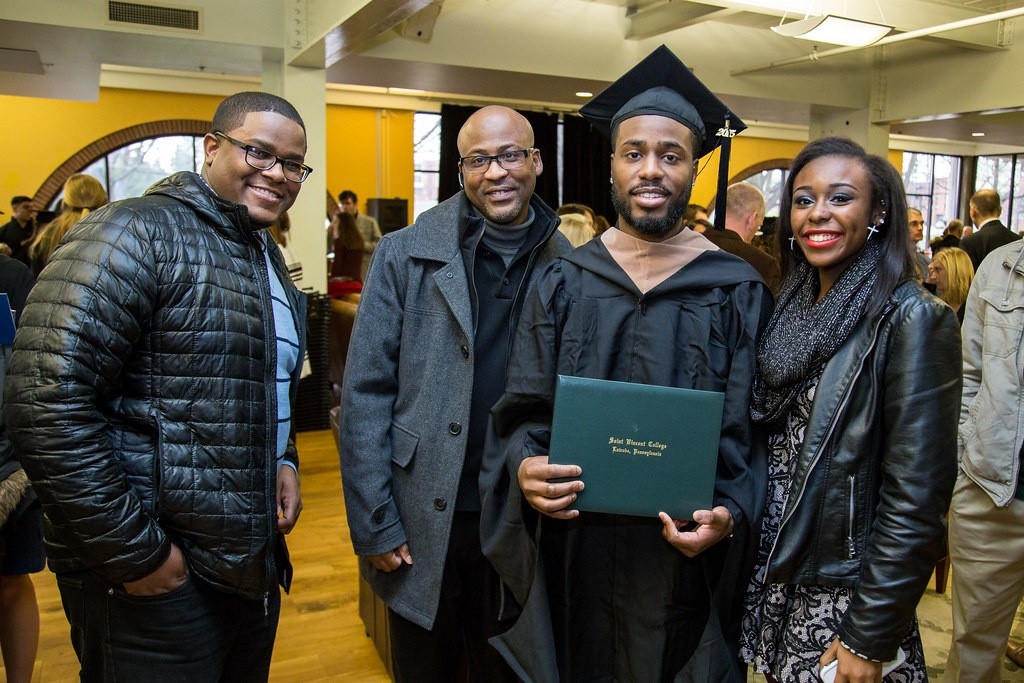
[1005,640,1024,668]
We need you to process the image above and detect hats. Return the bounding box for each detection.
[578,44,749,231]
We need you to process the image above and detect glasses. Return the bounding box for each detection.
[460,148,534,174]
[213,132,313,183]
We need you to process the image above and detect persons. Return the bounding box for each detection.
[473,43,773,683]
[327,190,382,282]
[703,180,781,302]
[331,212,364,281]
[729,136,965,682]
[904,188,1023,327]
[943,237,1024,683]
[682,203,708,234]
[338,105,575,682]
[555,204,609,249]
[0,93,313,683]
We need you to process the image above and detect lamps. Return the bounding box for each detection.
[770,0,896,48]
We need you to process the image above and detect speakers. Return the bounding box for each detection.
[365,197,408,236]
[392,0,444,44]
